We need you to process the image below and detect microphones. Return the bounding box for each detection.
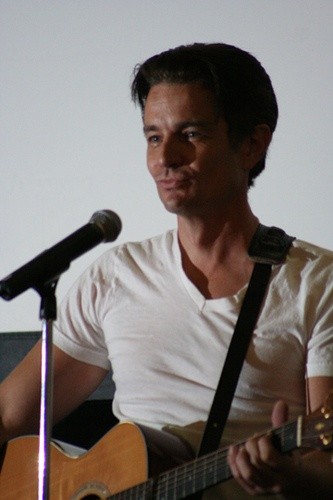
[0,209,122,302]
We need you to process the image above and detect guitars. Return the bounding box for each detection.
[0,390,333,500]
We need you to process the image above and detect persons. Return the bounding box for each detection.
[0,41,333,499]
